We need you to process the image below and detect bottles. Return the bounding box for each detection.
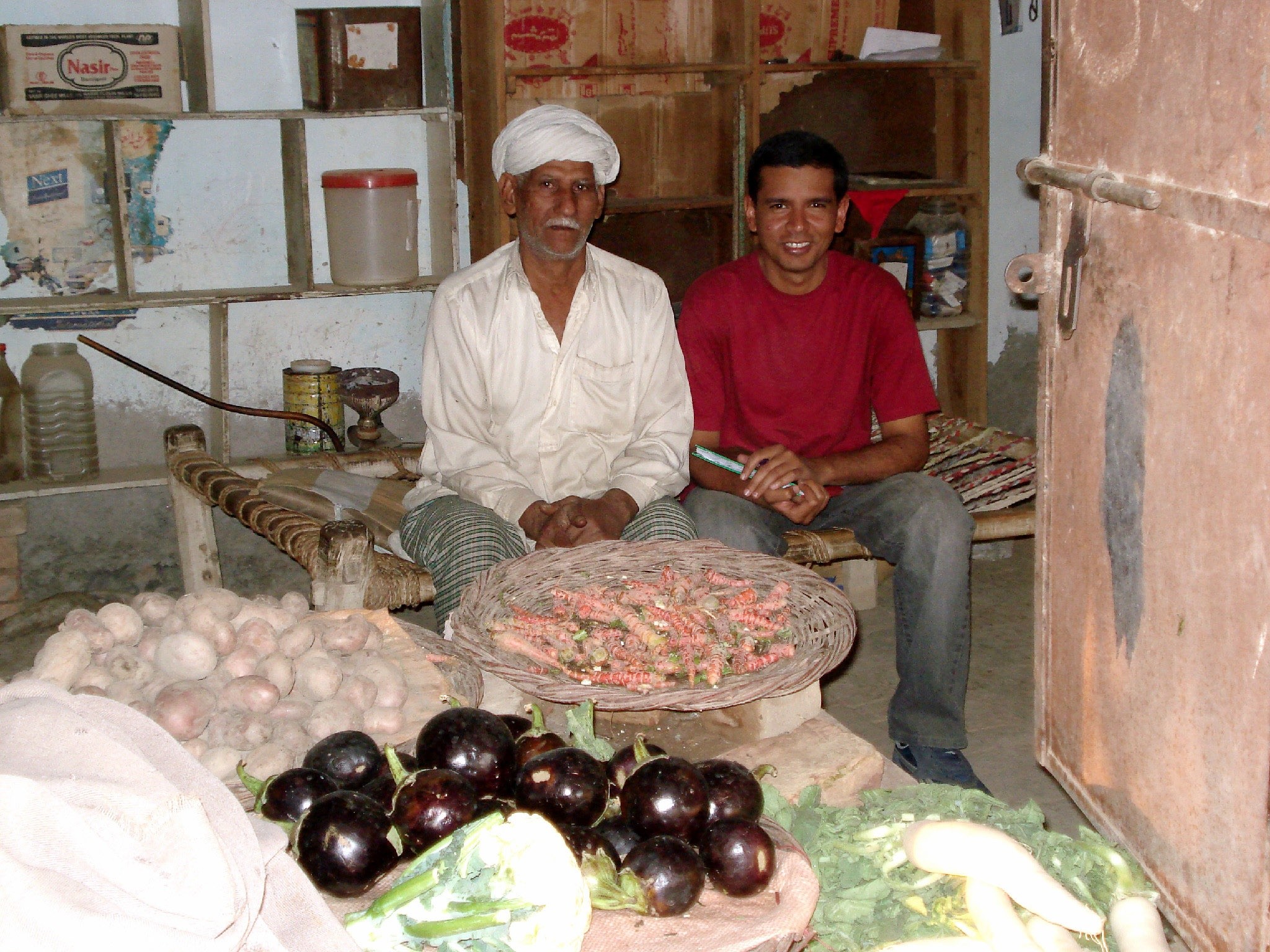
[906,198,970,317]
[0,343,24,484]
[282,359,344,453]
[321,168,419,285]
[19,343,100,484]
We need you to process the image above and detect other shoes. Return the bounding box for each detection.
[892,742,996,800]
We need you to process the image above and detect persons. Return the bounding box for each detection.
[675,131,994,796]
[398,105,698,639]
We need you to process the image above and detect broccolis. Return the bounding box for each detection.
[348,810,591,951]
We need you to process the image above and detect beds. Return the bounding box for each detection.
[159,402,1038,618]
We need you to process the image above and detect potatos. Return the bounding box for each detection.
[9,589,408,782]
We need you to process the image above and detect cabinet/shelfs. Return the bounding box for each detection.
[458,0,995,442]
[0,0,472,689]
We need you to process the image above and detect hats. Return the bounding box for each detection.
[492,103,621,186]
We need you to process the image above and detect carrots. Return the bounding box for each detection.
[483,565,793,692]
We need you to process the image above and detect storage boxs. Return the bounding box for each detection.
[0,21,186,121]
[0,337,103,494]
[828,199,973,326]
[294,4,426,114]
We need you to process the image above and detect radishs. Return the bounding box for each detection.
[902,820,1170,952]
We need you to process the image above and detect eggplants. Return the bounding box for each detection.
[235,696,775,914]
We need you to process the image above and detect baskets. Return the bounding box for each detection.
[451,539,857,711]
[223,610,485,813]
